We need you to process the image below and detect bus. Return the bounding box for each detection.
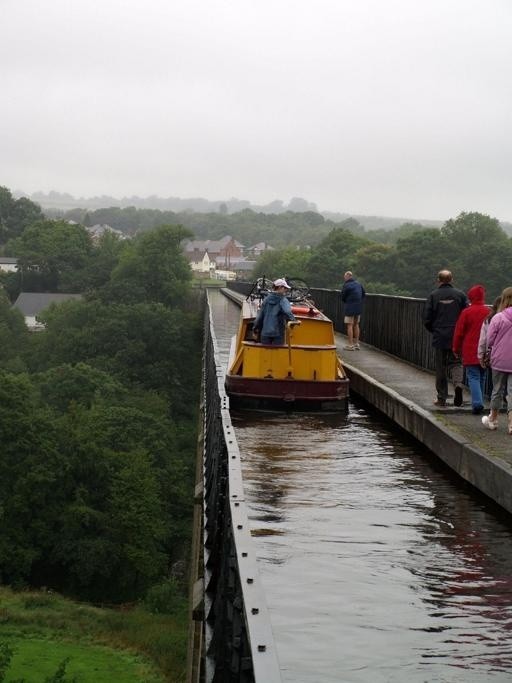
[215,270,237,281]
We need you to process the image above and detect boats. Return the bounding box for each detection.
[224,292,354,419]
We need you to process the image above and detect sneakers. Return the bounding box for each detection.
[343,343,359,351]
[453,385,462,406]
[481,415,498,430]
[473,405,484,414]
[508,424,512,434]
[433,399,446,406]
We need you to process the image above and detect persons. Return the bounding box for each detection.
[478,294,508,413]
[339,270,366,351]
[452,283,496,413]
[421,268,471,406]
[482,285,512,435]
[251,277,302,346]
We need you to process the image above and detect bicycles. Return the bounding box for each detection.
[243,276,311,302]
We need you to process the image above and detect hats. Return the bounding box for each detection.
[273,279,291,289]
